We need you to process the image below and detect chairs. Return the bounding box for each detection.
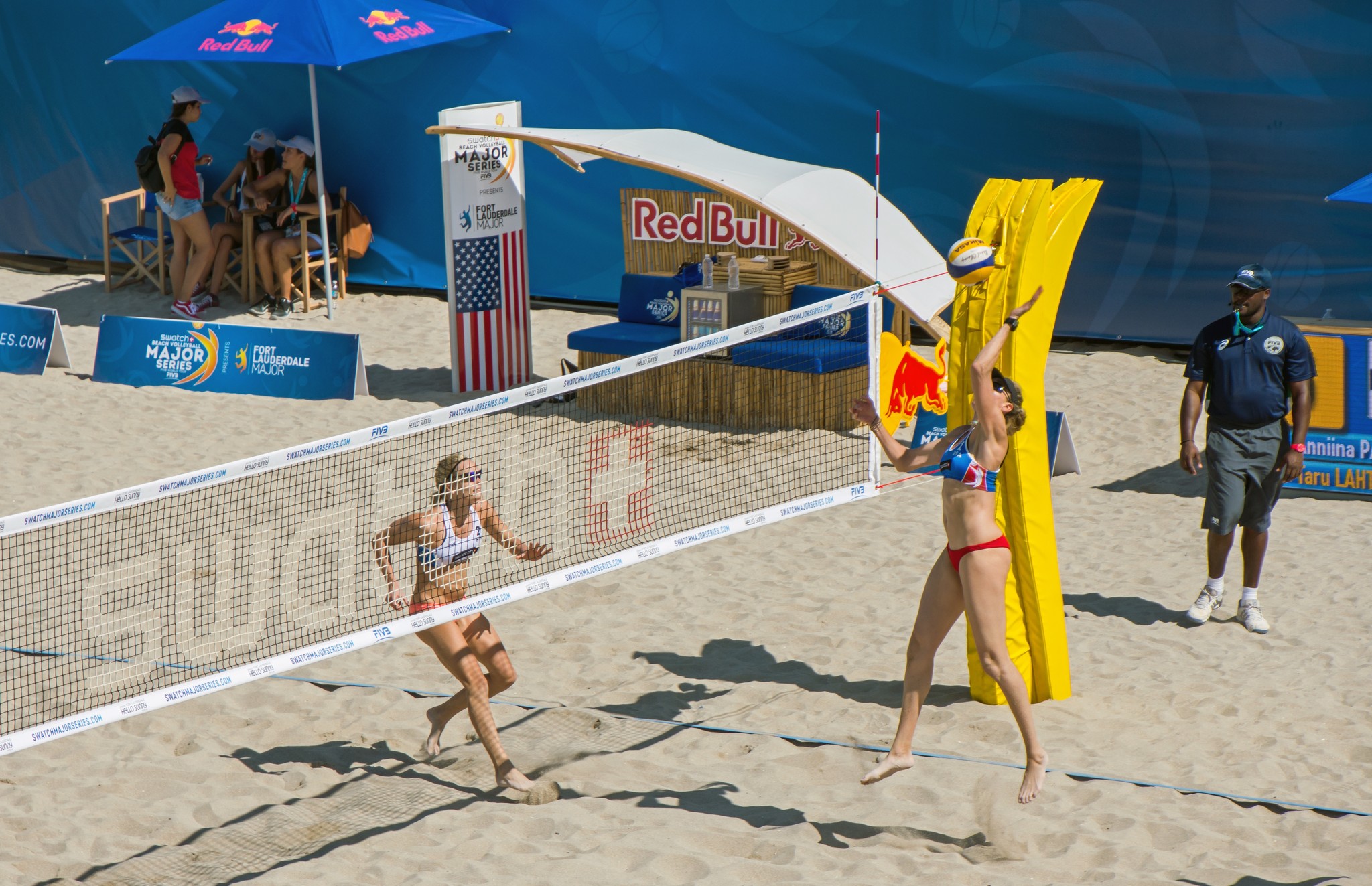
[100,173,346,314]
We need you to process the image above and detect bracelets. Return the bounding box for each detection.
[227,203,234,210]
[1180,439,1195,445]
[868,413,883,433]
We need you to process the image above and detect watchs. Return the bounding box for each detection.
[290,202,298,214]
[1002,317,1019,332]
[1290,443,1305,452]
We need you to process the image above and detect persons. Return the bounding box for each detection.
[1181,264,1317,634]
[372,454,555,792]
[243,135,332,320]
[155,85,214,322]
[190,127,279,309]
[848,285,1048,805]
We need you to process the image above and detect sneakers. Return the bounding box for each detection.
[190,282,206,301]
[195,294,220,309]
[171,300,202,321]
[1185,584,1224,624]
[270,296,295,319]
[1235,599,1270,634]
[247,292,276,314]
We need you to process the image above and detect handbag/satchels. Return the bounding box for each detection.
[337,192,375,258]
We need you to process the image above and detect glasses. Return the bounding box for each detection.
[993,381,1011,403]
[457,469,483,482]
[1231,285,1266,297]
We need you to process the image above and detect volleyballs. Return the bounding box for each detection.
[947,236,995,286]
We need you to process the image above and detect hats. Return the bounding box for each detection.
[276,135,314,157]
[1226,263,1273,290]
[992,366,1018,404]
[244,127,277,152]
[170,86,211,105]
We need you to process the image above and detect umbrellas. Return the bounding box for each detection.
[103,0,510,321]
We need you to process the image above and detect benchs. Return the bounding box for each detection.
[565,270,895,431]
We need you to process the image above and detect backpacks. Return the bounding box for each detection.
[134,121,186,194]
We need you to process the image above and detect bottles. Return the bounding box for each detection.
[702,254,714,288]
[332,280,339,310]
[1323,308,1335,319]
[728,256,739,289]
